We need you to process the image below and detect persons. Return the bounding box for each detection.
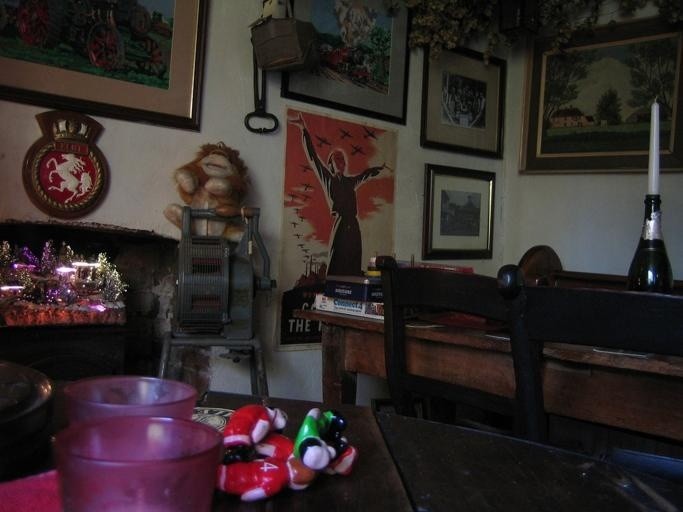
[291,113,388,278]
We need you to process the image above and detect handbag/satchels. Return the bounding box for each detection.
[250,15,321,73]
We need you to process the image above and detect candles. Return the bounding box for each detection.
[648,95,662,197]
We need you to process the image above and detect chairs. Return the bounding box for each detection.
[368,255,682,466]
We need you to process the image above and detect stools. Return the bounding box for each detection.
[159,328,278,398]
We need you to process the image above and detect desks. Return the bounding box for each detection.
[0,358,683,509]
[293,256,682,489]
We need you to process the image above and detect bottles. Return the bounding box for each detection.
[626,195,673,289]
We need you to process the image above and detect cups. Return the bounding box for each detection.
[49,378,217,512]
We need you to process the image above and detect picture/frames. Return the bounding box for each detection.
[422,162,498,263]
[277,0,411,130]
[518,2,683,179]
[2,2,215,138]
[417,40,507,160]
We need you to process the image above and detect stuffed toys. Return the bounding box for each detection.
[165,139,254,249]
